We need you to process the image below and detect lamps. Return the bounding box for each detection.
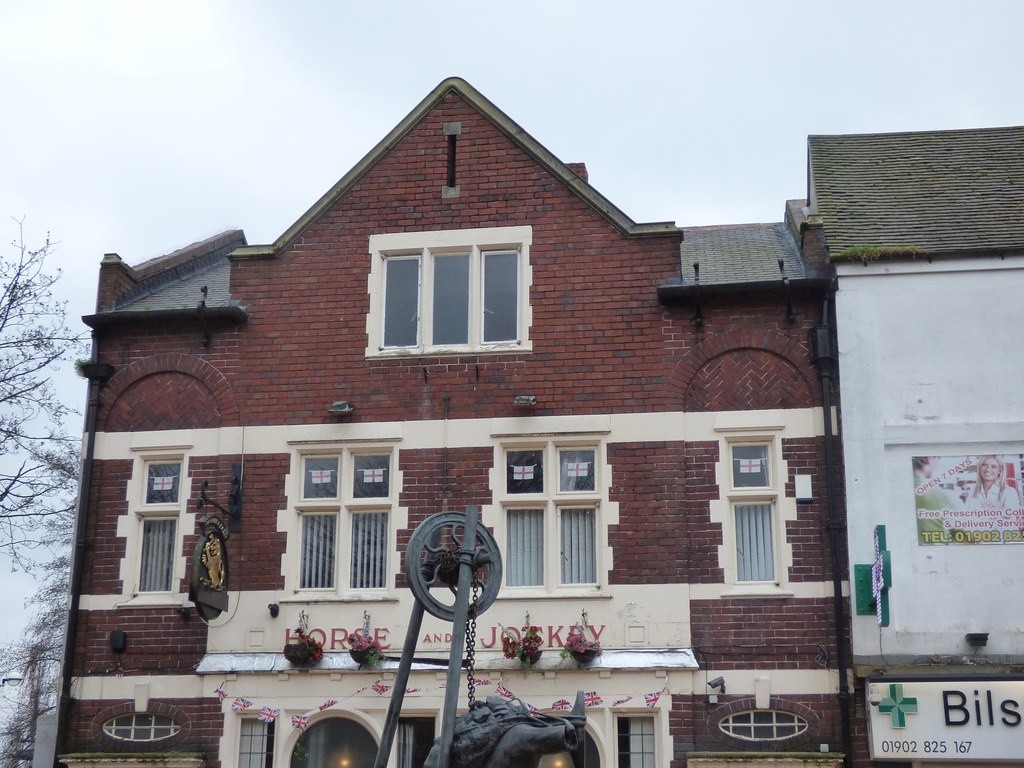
[328,401,354,413]
[513,396,536,406]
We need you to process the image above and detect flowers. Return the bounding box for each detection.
[559,622,600,661]
[347,633,391,673]
[503,626,544,679]
[283,628,323,662]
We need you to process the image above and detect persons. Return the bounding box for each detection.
[913,455,1019,544]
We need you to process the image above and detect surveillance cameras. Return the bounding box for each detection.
[707,676,725,689]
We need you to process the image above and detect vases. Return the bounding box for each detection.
[520,650,543,666]
[349,649,371,665]
[568,648,597,664]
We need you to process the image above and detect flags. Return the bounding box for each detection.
[215,680,663,730]
[739,460,761,473]
[153,477,172,490]
[513,463,588,480]
[311,468,384,484]
